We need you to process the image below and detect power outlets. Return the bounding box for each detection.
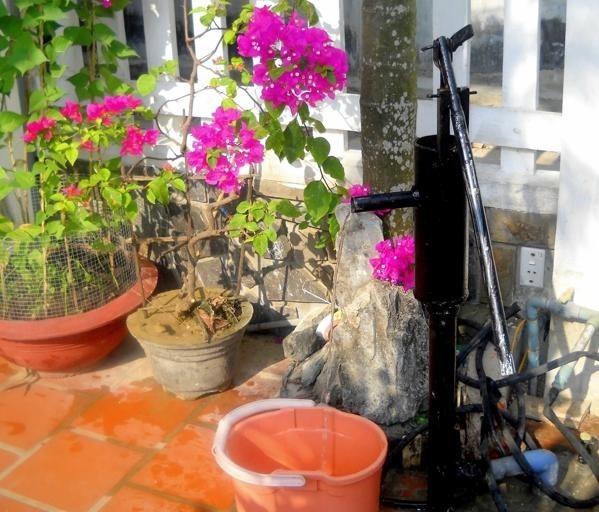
[518,247,546,290]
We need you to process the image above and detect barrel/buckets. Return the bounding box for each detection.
[209,395,389,511]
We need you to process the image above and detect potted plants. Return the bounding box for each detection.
[2,0,157,367]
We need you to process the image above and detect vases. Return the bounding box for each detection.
[127,287,251,399]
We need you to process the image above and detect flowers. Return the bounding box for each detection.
[33,9,418,309]
